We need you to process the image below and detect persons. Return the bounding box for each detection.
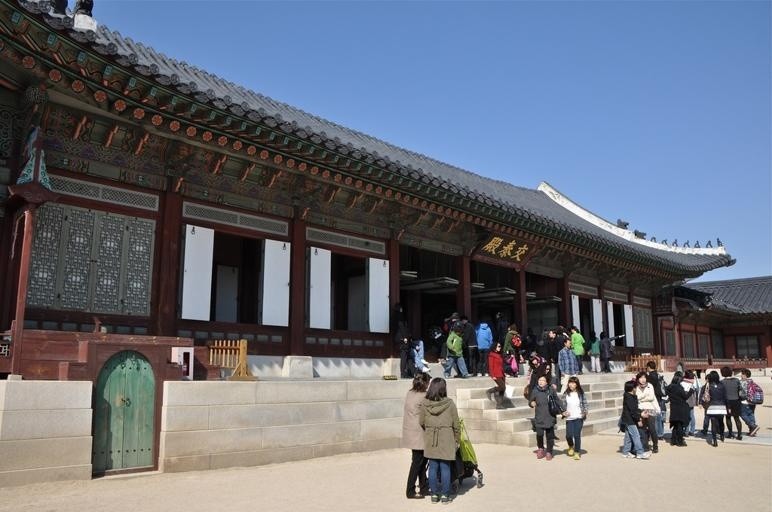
[390,302,433,380]
[419,377,461,504]
[401,372,431,499]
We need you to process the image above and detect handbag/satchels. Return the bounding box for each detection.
[511,357,519,372]
[504,383,515,400]
[701,383,711,403]
[549,388,561,415]
[524,385,530,399]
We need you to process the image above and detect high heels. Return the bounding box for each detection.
[712,433,743,446]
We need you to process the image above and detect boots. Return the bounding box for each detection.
[485,387,496,400]
[496,397,506,409]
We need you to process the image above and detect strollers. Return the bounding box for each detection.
[417,417,484,499]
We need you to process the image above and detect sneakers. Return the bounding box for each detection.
[747,432,757,437]
[623,437,663,459]
[701,430,707,435]
[749,425,760,436]
[443,372,489,378]
[574,451,581,460]
[671,432,695,447]
[536,448,545,459]
[546,451,553,460]
[568,445,574,456]
[407,489,449,504]
[421,358,429,365]
[422,367,430,373]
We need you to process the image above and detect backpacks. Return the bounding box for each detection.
[657,373,668,402]
[745,378,764,404]
[511,332,521,347]
